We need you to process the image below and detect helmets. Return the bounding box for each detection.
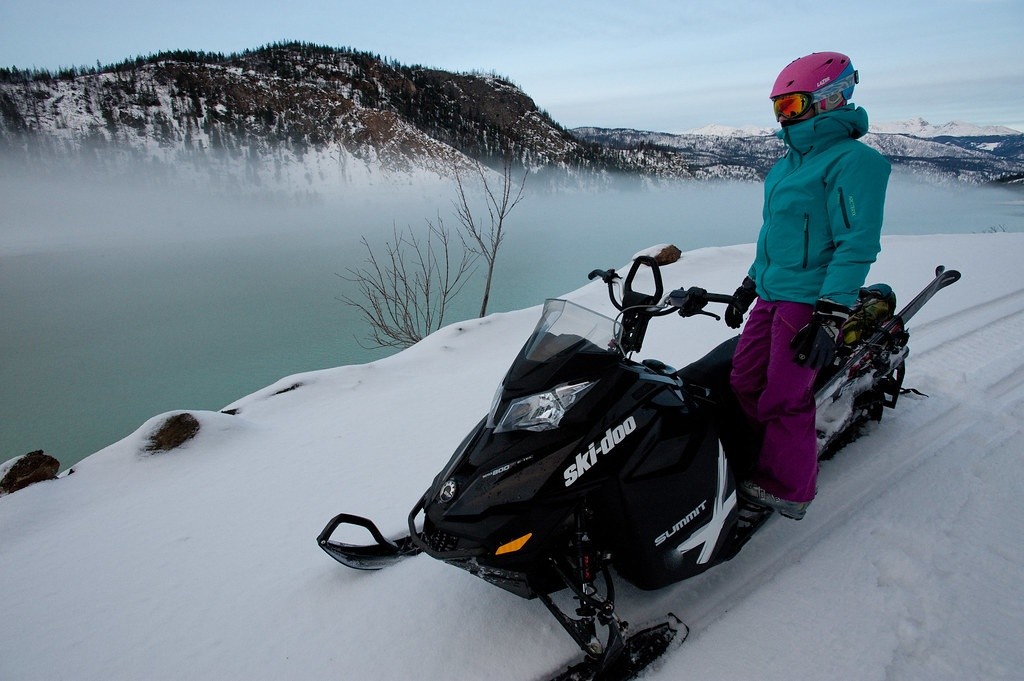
[769,52,856,114]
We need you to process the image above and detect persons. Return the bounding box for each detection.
[723,51,891,519]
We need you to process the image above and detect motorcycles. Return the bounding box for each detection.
[315,251,962,681]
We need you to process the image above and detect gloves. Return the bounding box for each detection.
[790,299,853,369]
[725,274,758,330]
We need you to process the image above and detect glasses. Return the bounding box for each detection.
[773,92,814,123]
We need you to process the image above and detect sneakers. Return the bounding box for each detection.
[739,479,812,520]
